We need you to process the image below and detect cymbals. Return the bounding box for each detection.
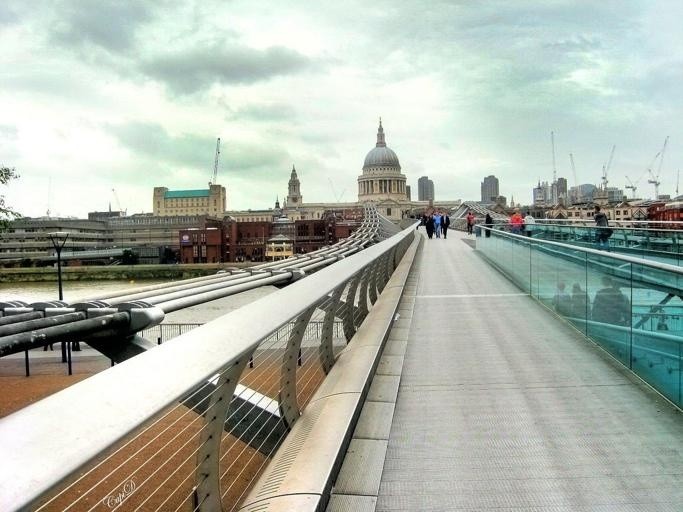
[550,131,681,198]
[211,137,223,185]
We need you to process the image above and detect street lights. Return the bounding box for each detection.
[606,228,613,237]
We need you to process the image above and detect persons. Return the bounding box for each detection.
[522,212,536,237]
[593,205,611,252]
[485,214,492,237]
[611,281,631,327]
[510,212,522,234]
[590,277,625,326]
[466,212,474,234]
[654,309,668,330]
[570,284,591,319]
[422,211,450,239]
[551,282,572,318]
